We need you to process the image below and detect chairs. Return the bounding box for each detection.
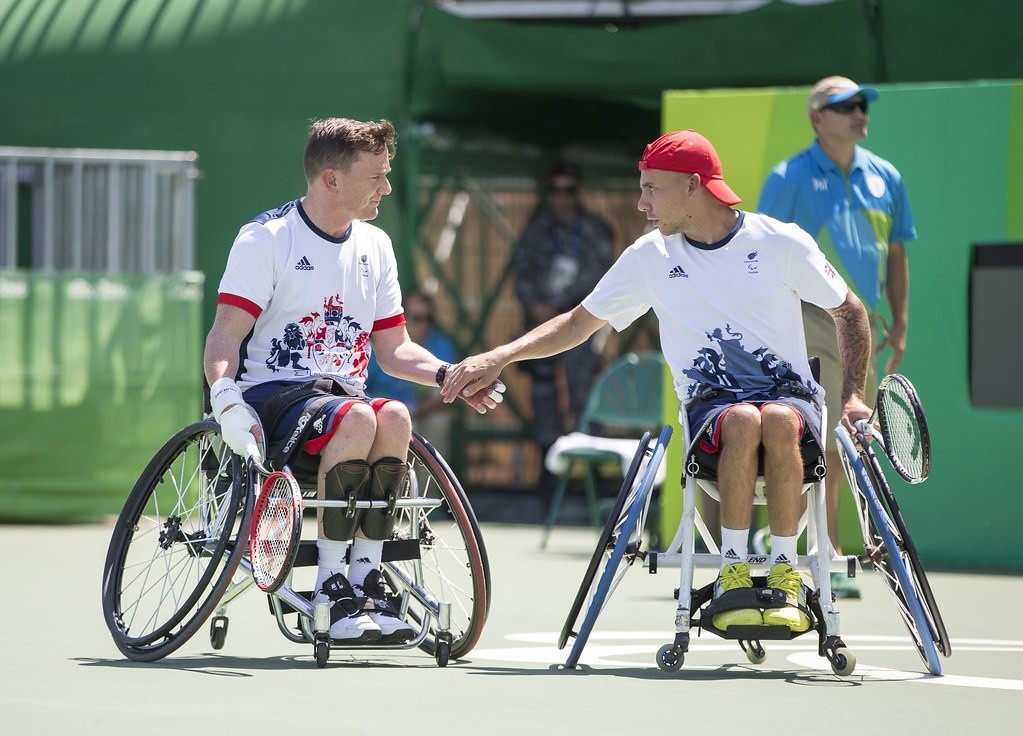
[540,355,668,553]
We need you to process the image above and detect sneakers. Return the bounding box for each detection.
[309,571,383,643]
[712,561,763,631]
[761,562,811,632]
[352,568,413,642]
[831,572,861,599]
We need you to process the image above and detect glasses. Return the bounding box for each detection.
[823,101,867,115]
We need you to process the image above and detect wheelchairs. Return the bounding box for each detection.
[101,322,492,669]
[557,356,952,678]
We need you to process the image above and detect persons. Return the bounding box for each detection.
[204,116,506,644]
[439,126,880,631]
[514,158,616,494]
[757,76,918,599]
[365,291,457,506]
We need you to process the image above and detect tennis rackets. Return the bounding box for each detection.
[246,454,302,592]
[856,374,930,483]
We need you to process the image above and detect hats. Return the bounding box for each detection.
[809,76,879,112]
[639,128,741,207]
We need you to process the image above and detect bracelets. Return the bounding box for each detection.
[436,364,453,390]
